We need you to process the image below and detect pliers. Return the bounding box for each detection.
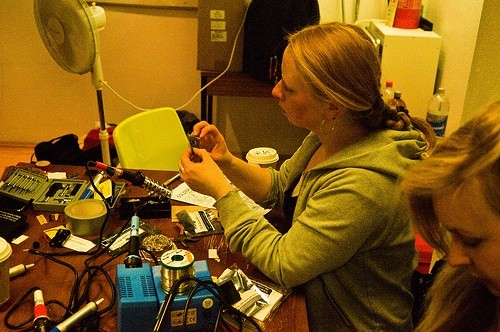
[169,223,202,249]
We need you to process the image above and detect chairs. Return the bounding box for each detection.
[112,107,192,171]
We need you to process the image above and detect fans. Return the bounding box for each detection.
[33,0,112,165]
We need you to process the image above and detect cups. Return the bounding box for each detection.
[246,147,280,169]
[0,236,12,306]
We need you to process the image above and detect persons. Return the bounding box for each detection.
[404,101,500,332]
[178,24,435,332]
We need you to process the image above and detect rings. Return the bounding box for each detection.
[178,166,185,174]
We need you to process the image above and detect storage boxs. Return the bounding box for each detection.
[196,0,247,72]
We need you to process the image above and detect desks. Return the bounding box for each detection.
[0,162,308,332]
[201,71,276,125]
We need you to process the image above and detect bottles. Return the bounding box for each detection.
[425,87,450,141]
[387,91,406,110]
[381,79,395,101]
